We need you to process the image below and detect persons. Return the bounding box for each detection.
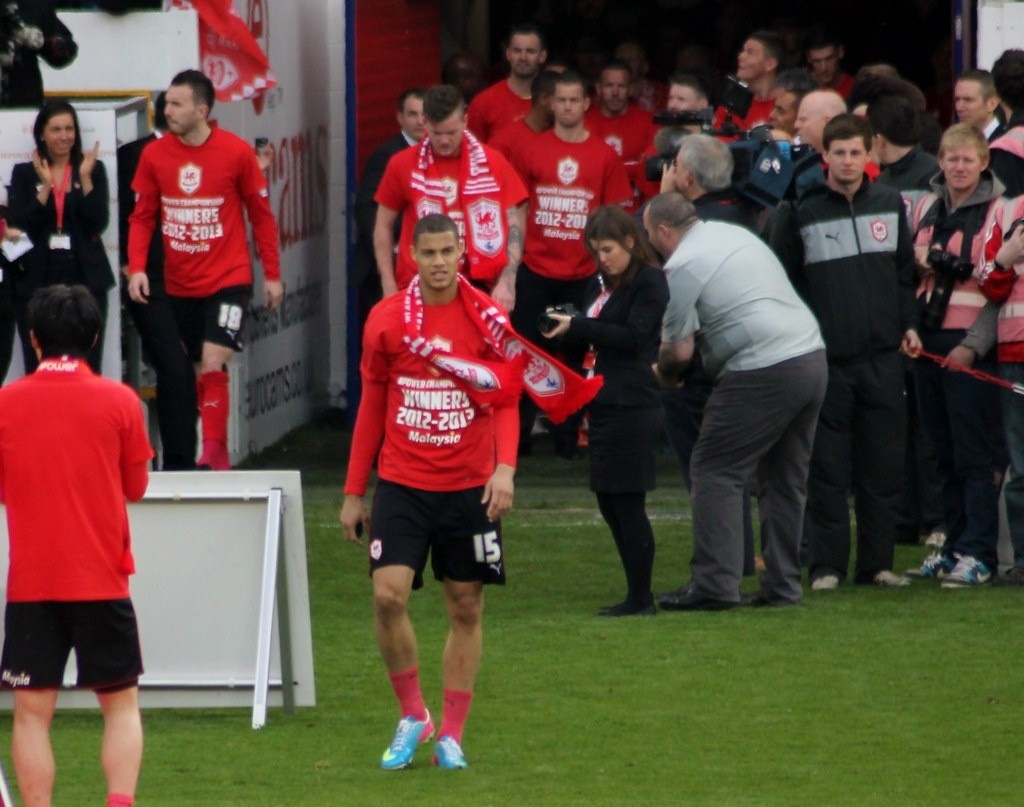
[0,101,116,385]
[643,193,827,609]
[117,91,274,471]
[543,203,670,615]
[356,23,665,458]
[126,70,283,470]
[636,23,1024,591]
[341,214,601,772]
[0,0,77,106]
[0,286,155,807]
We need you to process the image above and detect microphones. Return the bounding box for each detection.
[654,127,693,156]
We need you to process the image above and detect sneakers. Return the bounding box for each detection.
[941,552,991,587]
[907,549,949,576]
[381,707,435,771]
[431,735,469,769]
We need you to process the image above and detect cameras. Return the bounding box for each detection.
[535,303,579,333]
[921,247,974,333]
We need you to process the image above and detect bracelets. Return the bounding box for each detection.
[994,259,1008,270]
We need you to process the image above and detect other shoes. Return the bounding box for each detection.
[598,598,656,618]
[812,574,838,588]
[873,569,910,587]
[518,437,542,458]
[659,580,735,611]
[547,434,585,459]
[739,588,797,606]
[924,530,947,547]
